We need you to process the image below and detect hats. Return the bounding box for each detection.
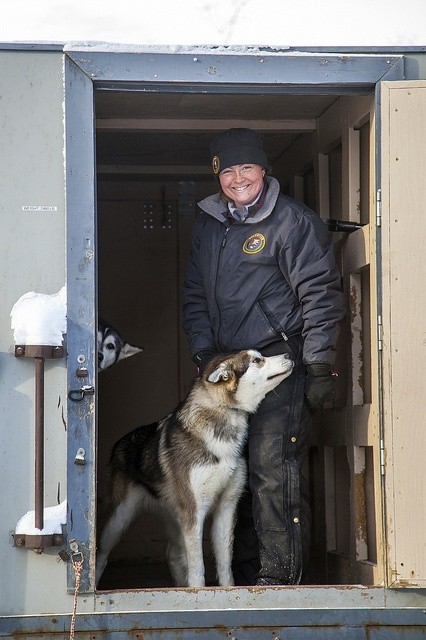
[209,128,272,177]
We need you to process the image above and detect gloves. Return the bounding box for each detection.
[193,352,215,375]
[303,364,335,414]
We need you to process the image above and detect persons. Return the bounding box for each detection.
[181,128,343,586]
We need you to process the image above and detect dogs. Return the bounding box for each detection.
[96,324,144,373]
[95,347,297,591]
